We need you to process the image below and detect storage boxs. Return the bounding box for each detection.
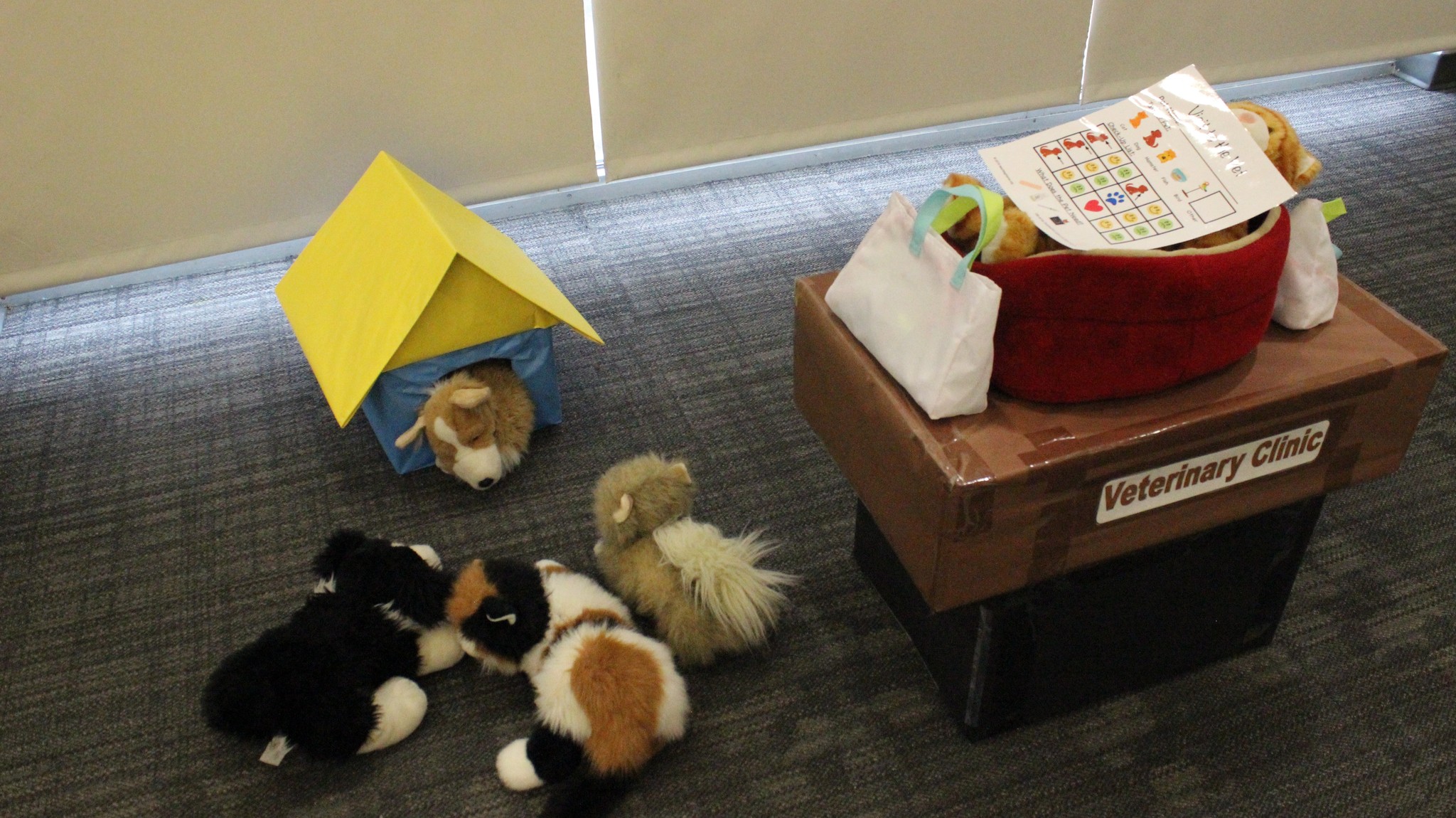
[793,269,1445,740]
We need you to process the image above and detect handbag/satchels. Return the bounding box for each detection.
[825,183,1004,421]
[1270,195,1348,331]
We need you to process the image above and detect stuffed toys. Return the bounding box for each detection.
[935,96,1322,266]
[591,451,800,671]
[394,361,536,491]
[446,557,693,818]
[201,526,464,766]
